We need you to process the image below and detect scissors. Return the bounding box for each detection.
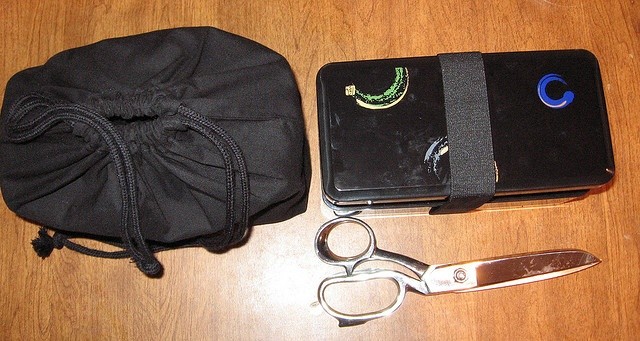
[315,216,601,327]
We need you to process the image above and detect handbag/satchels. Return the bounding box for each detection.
[1,26,312,277]
